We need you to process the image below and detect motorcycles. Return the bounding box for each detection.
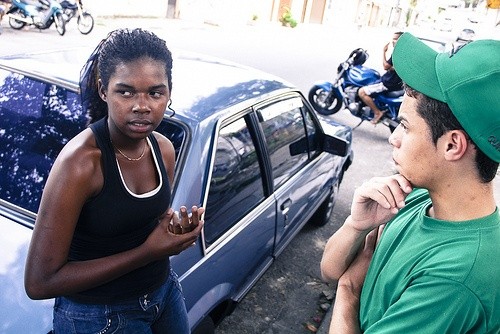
[307,48,402,134]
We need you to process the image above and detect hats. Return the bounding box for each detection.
[391,32,500,162]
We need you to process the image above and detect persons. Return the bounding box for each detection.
[358,32,403,123]
[320,32,500,334]
[23,28,204,334]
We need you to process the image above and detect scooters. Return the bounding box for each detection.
[0,0,94,36]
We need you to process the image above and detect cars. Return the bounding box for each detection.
[0,43,354,334]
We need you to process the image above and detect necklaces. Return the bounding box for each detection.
[109,137,146,160]
